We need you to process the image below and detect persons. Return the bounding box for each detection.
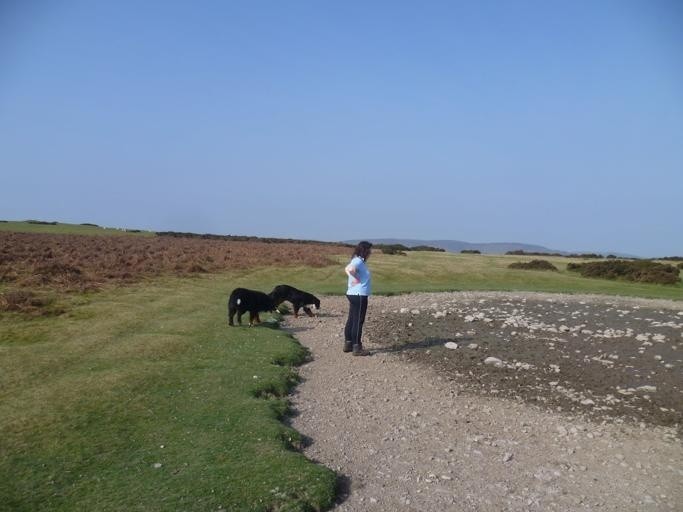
[343,241,372,356]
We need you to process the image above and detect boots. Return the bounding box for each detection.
[353,343,368,356]
[344,341,353,352]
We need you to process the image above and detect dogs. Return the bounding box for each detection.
[267,285,320,319]
[228,287,278,327]
